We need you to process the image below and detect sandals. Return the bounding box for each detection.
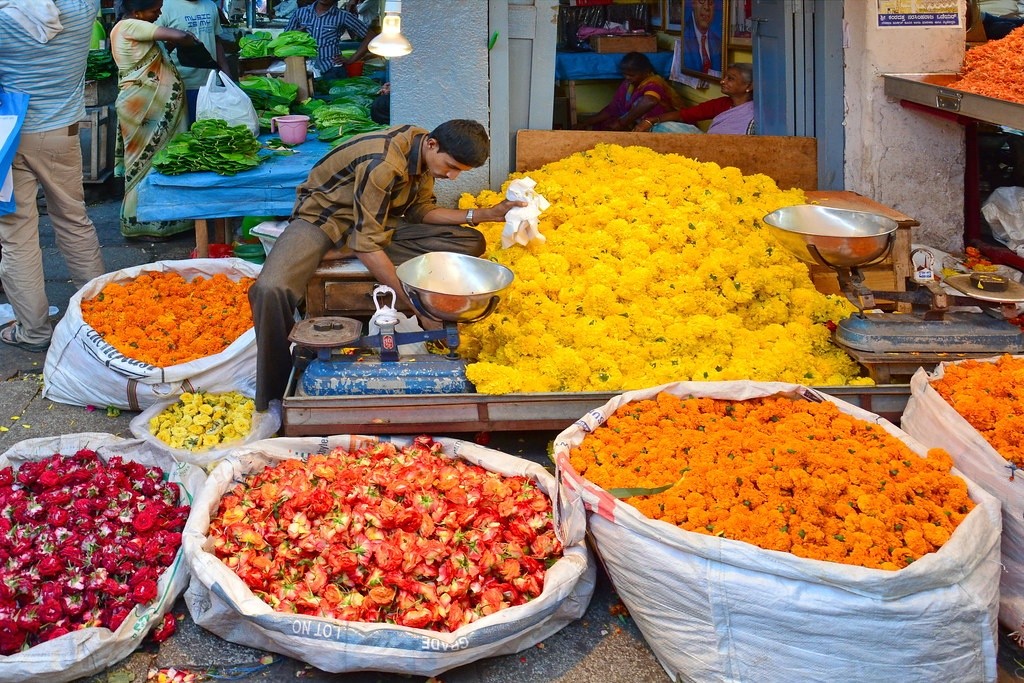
[0,322,51,351]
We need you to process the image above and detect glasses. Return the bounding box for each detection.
[699,0,714,4]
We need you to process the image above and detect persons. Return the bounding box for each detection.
[565,54,757,136]
[89,19,107,50]
[248,119,529,436]
[152,0,379,130]
[109,0,202,241]
[0,0,106,352]
[371,82,391,125]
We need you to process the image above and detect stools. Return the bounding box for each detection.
[304,258,413,313]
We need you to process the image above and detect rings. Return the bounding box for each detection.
[632,129,634,132]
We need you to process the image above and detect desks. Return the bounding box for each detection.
[556,51,672,128]
[136,132,336,257]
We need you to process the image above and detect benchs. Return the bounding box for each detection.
[642,47,755,134]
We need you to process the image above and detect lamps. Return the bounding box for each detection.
[367,0,413,56]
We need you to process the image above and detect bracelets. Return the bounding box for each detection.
[466,208,479,227]
[618,118,624,129]
[643,119,654,127]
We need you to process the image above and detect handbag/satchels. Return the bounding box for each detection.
[0,84,30,215]
[196,70,260,138]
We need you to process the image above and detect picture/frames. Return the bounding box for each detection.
[681,0,729,81]
[664,0,680,35]
[650,0,664,30]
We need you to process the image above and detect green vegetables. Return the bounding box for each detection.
[235,31,392,148]
[150,119,270,177]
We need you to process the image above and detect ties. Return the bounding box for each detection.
[701,35,710,74]
